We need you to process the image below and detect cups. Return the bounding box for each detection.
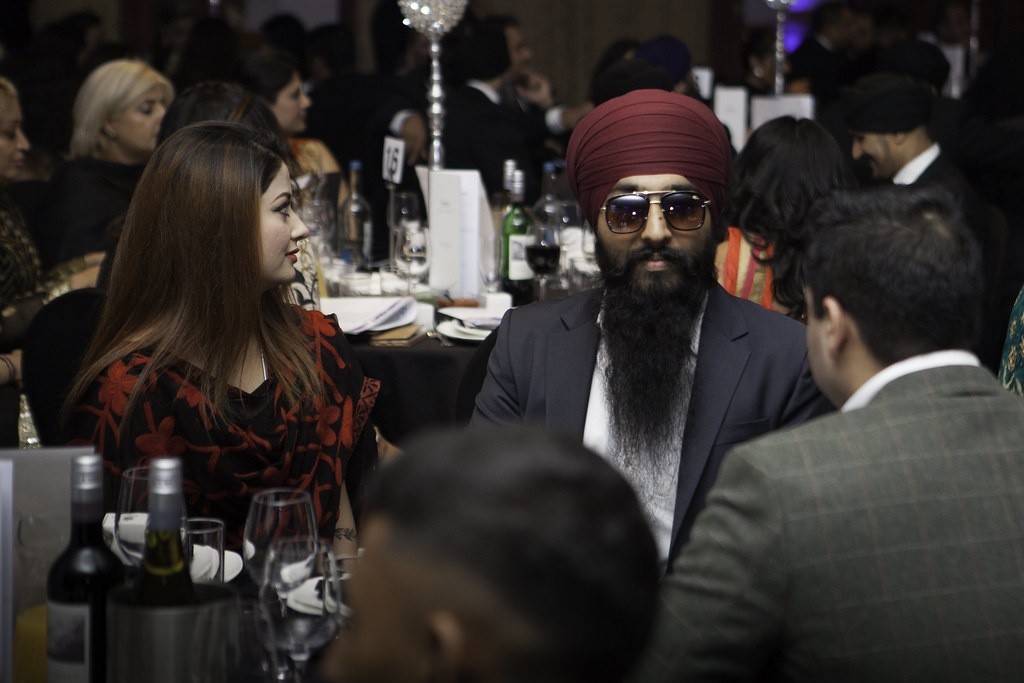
[12,520,72,683]
[319,544,364,639]
[183,516,226,588]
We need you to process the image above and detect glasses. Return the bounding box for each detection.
[597,189,711,235]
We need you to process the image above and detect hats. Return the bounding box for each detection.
[568,89,732,231]
[638,35,695,82]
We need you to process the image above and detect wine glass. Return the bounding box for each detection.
[256,539,339,683]
[284,188,431,304]
[241,488,318,679]
[523,216,605,301]
[114,465,187,575]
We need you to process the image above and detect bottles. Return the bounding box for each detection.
[44,456,126,683]
[532,160,566,247]
[137,457,202,611]
[340,160,373,269]
[492,158,542,308]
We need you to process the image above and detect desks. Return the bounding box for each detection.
[324,206,606,444]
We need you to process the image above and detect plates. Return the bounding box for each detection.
[437,317,501,341]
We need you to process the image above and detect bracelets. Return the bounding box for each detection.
[0,355,12,385]
[4,355,16,385]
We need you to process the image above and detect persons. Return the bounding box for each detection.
[621,187,1024,683]
[463,88,840,584]
[324,425,660,683]
[0,0,1024,453]
[56,121,382,588]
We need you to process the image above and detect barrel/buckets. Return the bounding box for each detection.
[97,590,249,682]
